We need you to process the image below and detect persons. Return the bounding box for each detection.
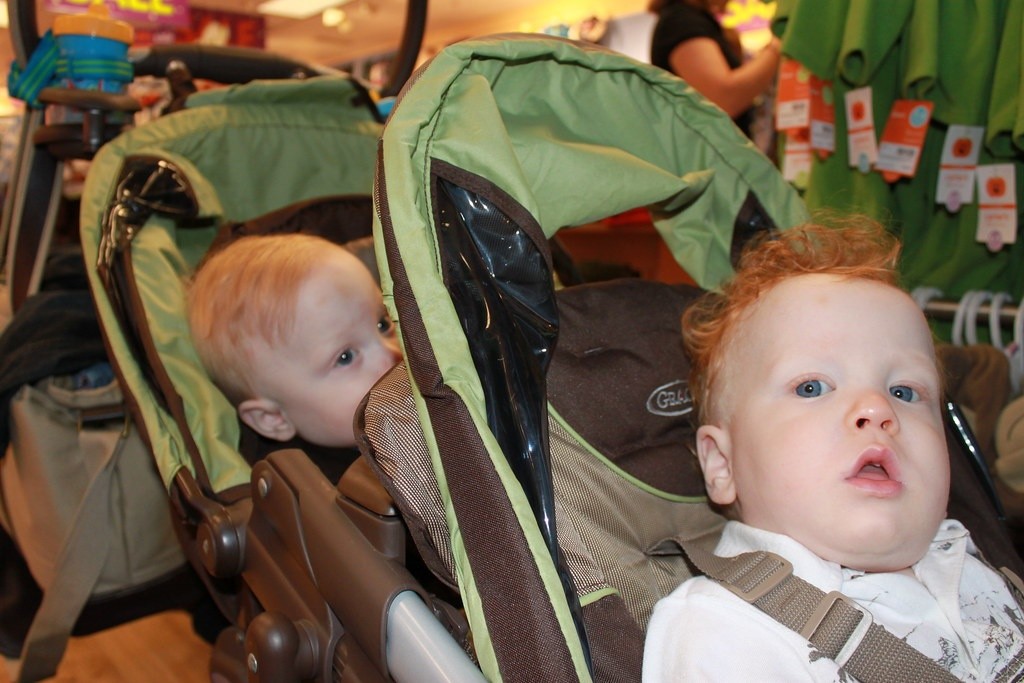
[650,0,781,139]
[192,234,404,484]
[640,224,1024,683]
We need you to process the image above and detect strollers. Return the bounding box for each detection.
[0,0,1024,683]
